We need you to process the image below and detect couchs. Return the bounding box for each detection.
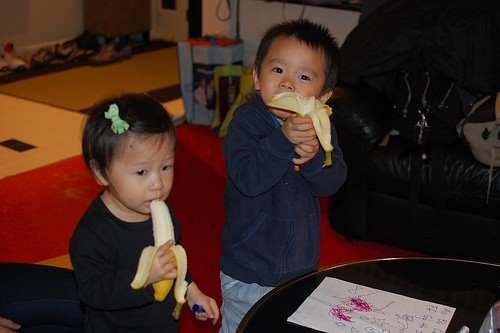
[329,0,500,263]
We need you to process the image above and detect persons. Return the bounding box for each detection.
[194,76,217,112]
[218,20,348,333]
[69,94,219,333]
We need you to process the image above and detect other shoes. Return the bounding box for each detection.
[31,31,132,71]
[0,57,9,73]
[3,52,27,72]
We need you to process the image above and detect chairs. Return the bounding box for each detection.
[0,261,82,333]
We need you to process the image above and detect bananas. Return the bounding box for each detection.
[268,92,334,167]
[130,200,189,320]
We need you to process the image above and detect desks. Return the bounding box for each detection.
[236,258,500,333]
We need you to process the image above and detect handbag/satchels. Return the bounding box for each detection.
[177,35,243,127]
[382,66,464,237]
